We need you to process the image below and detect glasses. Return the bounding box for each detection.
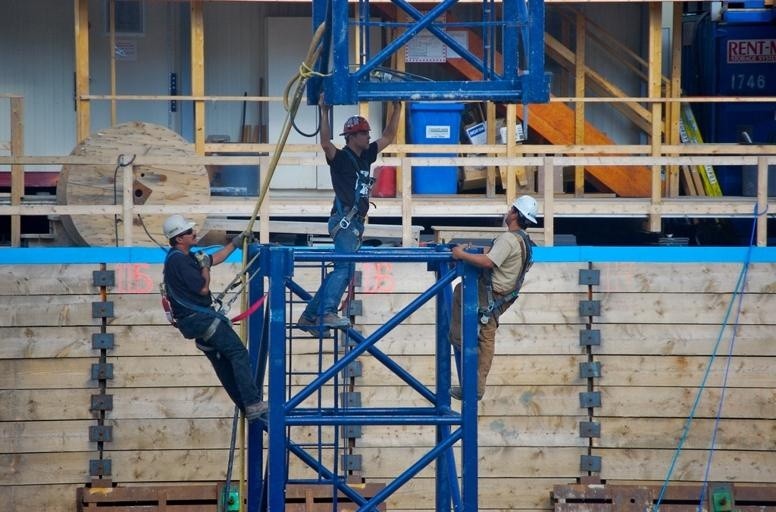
[174,229,190,238]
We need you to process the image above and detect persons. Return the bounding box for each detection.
[448,194,539,401]
[296,92,401,338]
[161,215,270,420]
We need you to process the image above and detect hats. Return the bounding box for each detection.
[512,195,538,224]
[164,215,196,239]
[339,115,371,135]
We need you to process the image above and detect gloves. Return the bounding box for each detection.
[232,230,253,246]
[194,252,210,268]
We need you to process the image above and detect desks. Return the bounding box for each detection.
[431,226,545,245]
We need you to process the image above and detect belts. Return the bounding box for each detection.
[335,205,366,226]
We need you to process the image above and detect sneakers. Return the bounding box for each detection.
[449,387,482,400]
[316,313,350,329]
[297,315,331,337]
[246,401,269,420]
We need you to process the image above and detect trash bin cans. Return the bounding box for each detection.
[407,101,465,195]
